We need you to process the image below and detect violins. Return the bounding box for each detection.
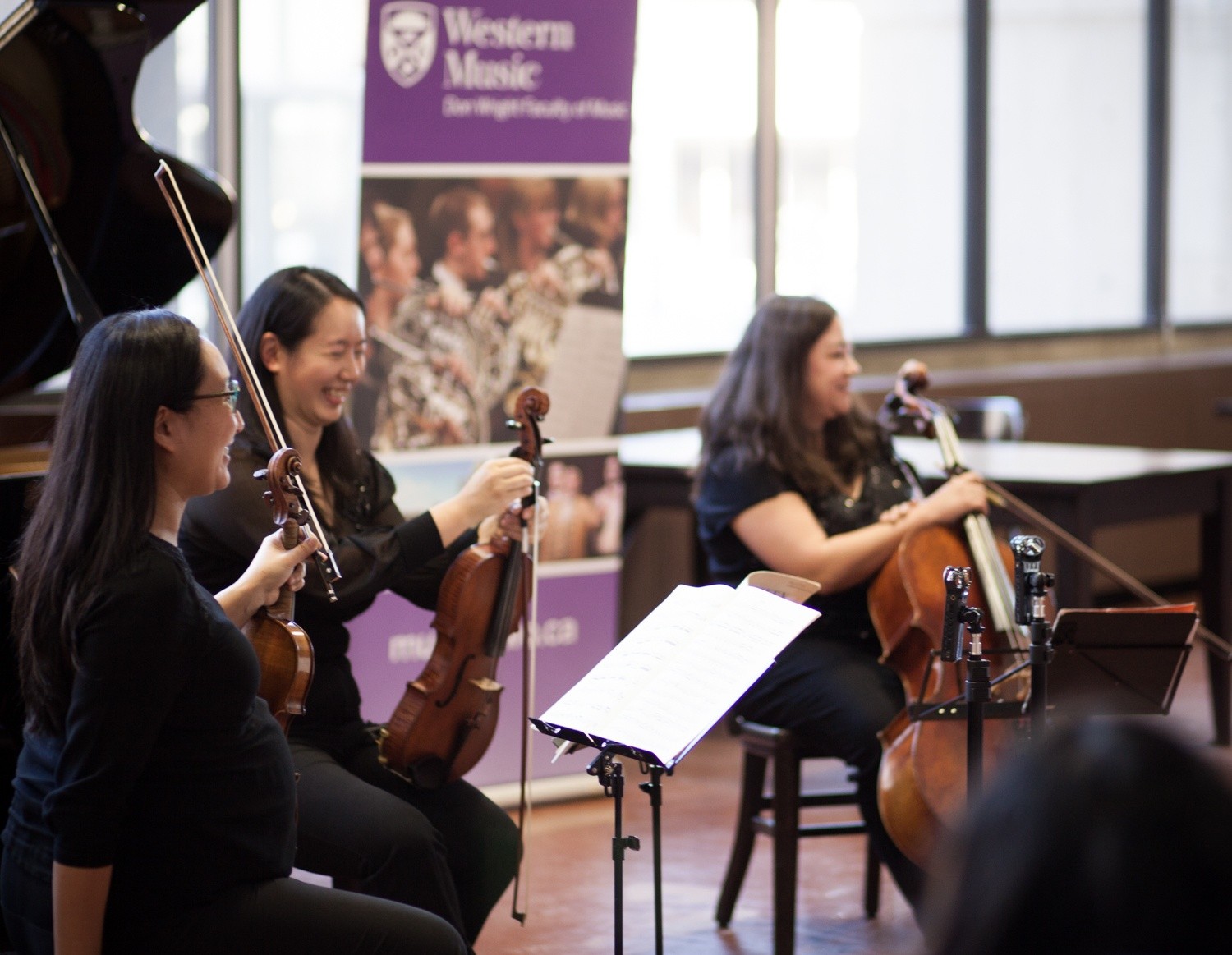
[237,448,314,733]
[373,386,555,786]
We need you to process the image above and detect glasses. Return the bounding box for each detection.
[192,379,241,414]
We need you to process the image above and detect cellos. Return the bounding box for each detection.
[867,356,1060,883]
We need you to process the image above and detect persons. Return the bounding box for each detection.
[530,460,629,561]
[0,310,470,955]
[692,293,1006,930]
[363,177,620,452]
[928,706,1232,955]
[177,268,550,955]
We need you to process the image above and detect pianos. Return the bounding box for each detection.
[0,0,237,844]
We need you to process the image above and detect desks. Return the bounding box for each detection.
[625,433,1232,748]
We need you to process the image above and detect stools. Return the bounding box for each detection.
[715,715,881,955]
